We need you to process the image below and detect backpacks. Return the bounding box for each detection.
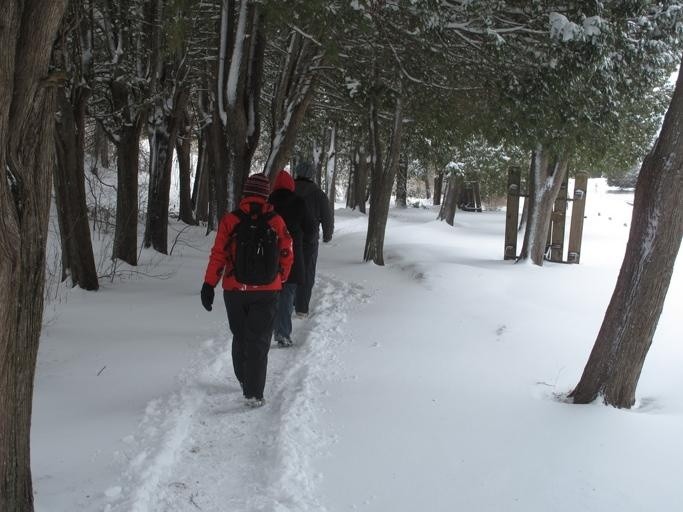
[228,207,280,285]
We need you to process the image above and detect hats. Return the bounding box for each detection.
[271,168,296,191]
[297,161,314,179]
[241,172,271,199]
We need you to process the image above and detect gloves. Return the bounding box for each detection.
[200,282,214,311]
[322,234,331,243]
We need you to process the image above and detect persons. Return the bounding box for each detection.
[201,173,293,408]
[268,170,313,347]
[294,161,334,317]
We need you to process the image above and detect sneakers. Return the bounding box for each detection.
[272,332,293,347]
[244,395,267,409]
[296,310,310,319]
